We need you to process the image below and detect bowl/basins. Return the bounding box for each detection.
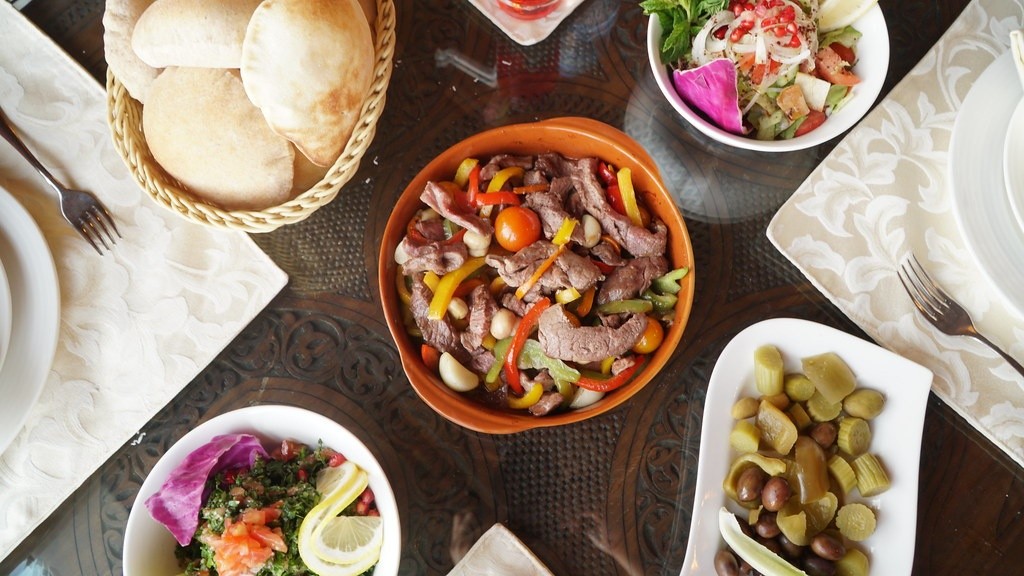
[123,405,402,576]
[647,0,890,152]
[1002,98,1024,232]
[378,115,694,434]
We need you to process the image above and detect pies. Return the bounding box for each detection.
[101,1,374,208]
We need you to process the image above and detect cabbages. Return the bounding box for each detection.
[738,64,853,140]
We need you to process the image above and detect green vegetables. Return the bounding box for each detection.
[640,0,728,64]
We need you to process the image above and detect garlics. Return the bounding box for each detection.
[583,214,602,247]
[572,386,605,409]
[440,351,479,391]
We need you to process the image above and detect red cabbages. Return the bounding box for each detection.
[676,59,745,131]
[142,433,266,547]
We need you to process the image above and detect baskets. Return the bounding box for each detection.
[105,0,397,232]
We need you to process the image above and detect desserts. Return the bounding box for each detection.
[498,0,564,19]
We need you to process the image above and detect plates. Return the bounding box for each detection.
[468,0,584,47]
[679,316,934,576]
[0,184,60,460]
[947,47,1024,318]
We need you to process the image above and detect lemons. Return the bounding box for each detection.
[297,463,385,576]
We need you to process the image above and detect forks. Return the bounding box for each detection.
[0,117,121,256]
[896,253,1024,378]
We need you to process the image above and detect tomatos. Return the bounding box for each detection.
[794,111,826,137]
[496,207,541,249]
[816,48,861,84]
[201,501,288,576]
[830,42,855,64]
